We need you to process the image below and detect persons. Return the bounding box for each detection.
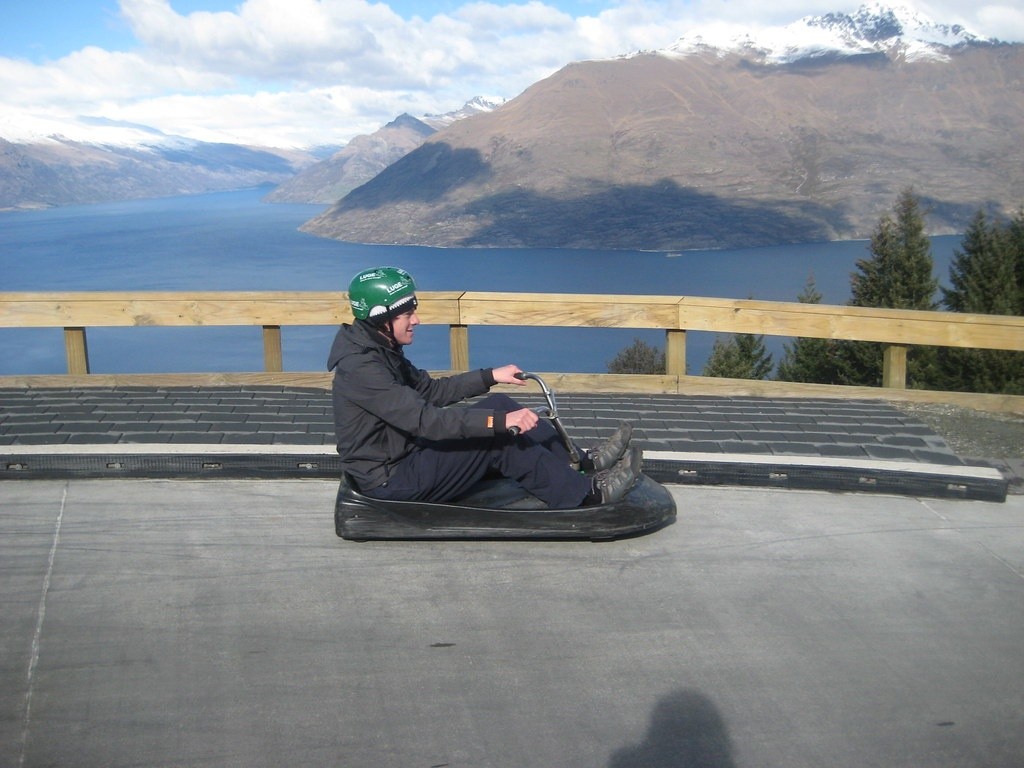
[327,267,643,507]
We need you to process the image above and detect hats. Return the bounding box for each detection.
[369,290,418,324]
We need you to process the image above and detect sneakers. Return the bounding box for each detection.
[592,446,644,505]
[587,421,634,473]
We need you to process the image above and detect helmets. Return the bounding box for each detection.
[348,266,417,321]
